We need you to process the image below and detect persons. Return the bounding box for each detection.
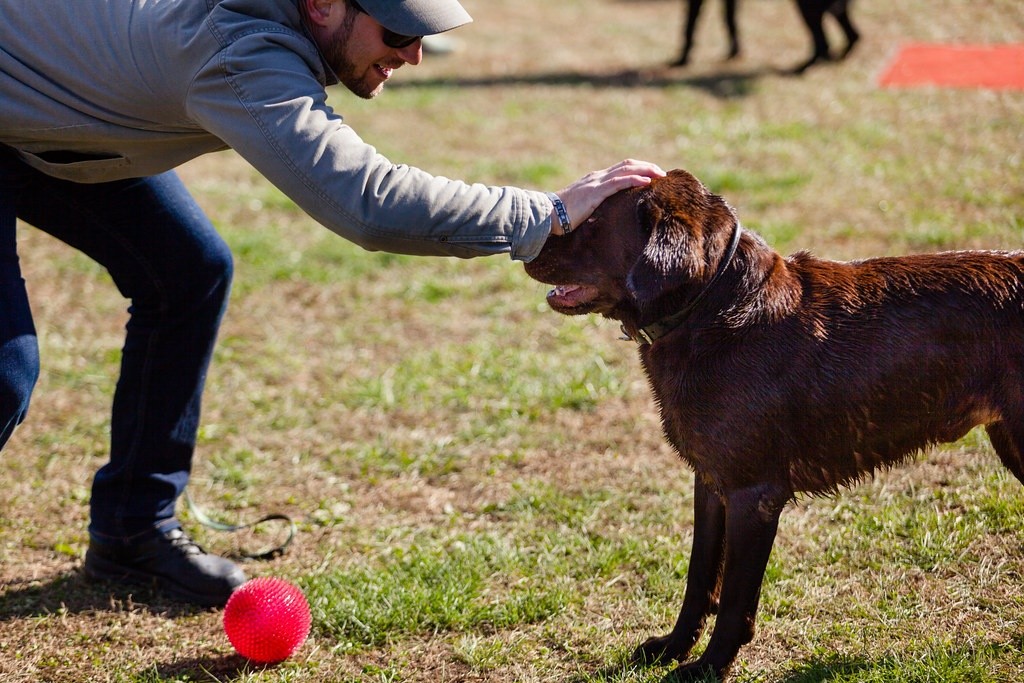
[0,0,666,609]
[671,0,739,66]
[794,0,859,74]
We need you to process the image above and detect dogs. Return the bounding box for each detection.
[522,169,1024,683]
[670,0,860,75]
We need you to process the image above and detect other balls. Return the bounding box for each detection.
[224,576,313,665]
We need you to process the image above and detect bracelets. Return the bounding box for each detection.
[546,192,572,234]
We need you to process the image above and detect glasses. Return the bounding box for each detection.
[381,28,424,49]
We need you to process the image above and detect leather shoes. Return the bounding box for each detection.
[84,526,244,608]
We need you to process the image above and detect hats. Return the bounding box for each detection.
[357,0,473,37]
